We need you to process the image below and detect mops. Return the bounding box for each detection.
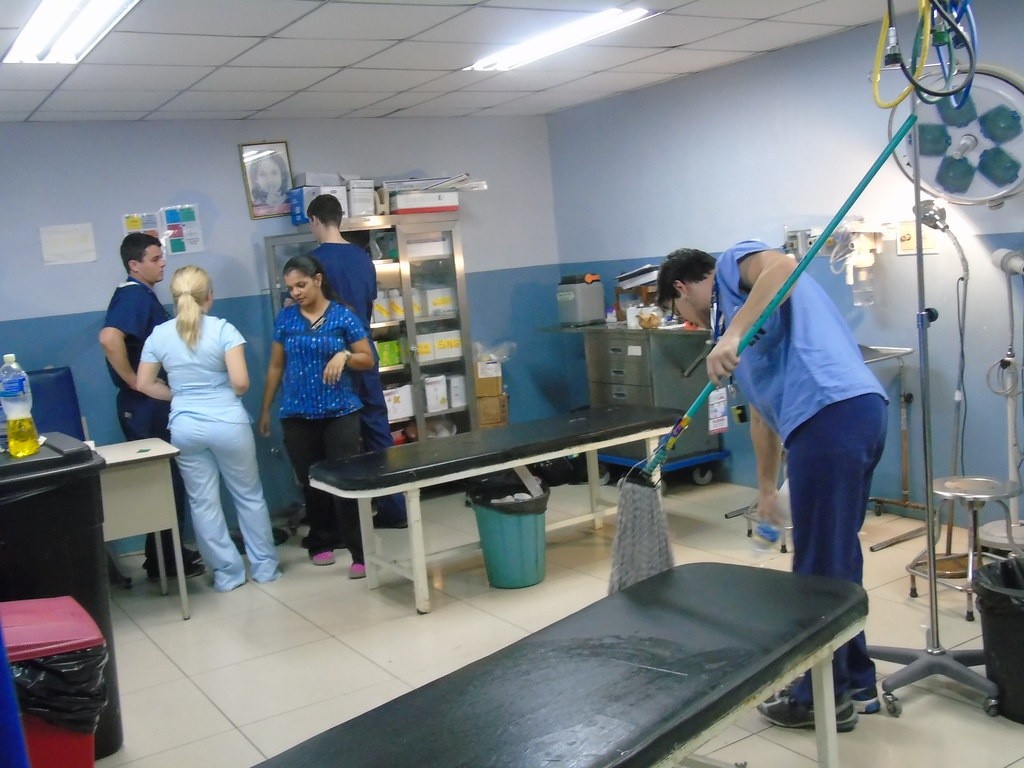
[606,112,920,598]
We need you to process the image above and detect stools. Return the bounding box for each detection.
[905,475,1023,620]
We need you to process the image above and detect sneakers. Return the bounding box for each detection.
[786,675,880,714]
[755,689,858,732]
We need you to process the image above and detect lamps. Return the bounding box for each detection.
[911,199,986,578]
[977,246,1024,552]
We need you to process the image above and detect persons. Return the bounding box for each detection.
[657,239,891,733]
[136,266,283,592]
[304,194,409,529]
[249,150,290,207]
[97,232,207,580]
[258,254,375,579]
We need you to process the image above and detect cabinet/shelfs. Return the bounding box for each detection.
[579,327,725,463]
[267,222,476,494]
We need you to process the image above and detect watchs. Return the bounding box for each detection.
[343,349,352,360]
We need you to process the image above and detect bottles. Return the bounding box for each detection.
[0,354,41,457]
[750,491,791,558]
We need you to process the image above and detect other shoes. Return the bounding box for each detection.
[312,549,336,565]
[348,556,367,579]
[373,513,407,529]
[172,545,200,561]
[146,560,205,582]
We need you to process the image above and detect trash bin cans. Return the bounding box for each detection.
[0,596,105,768]
[970,556,1023,725]
[468,477,550,589]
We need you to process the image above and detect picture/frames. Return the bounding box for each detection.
[238,140,295,220]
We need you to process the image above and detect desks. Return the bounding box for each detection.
[256,557,872,767]
[91,437,192,623]
[305,403,684,613]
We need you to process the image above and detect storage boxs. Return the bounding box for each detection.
[289,165,490,225]
[345,243,517,446]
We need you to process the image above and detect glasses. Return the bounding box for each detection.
[666,279,684,323]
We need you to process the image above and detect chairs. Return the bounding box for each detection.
[22,364,93,451]
